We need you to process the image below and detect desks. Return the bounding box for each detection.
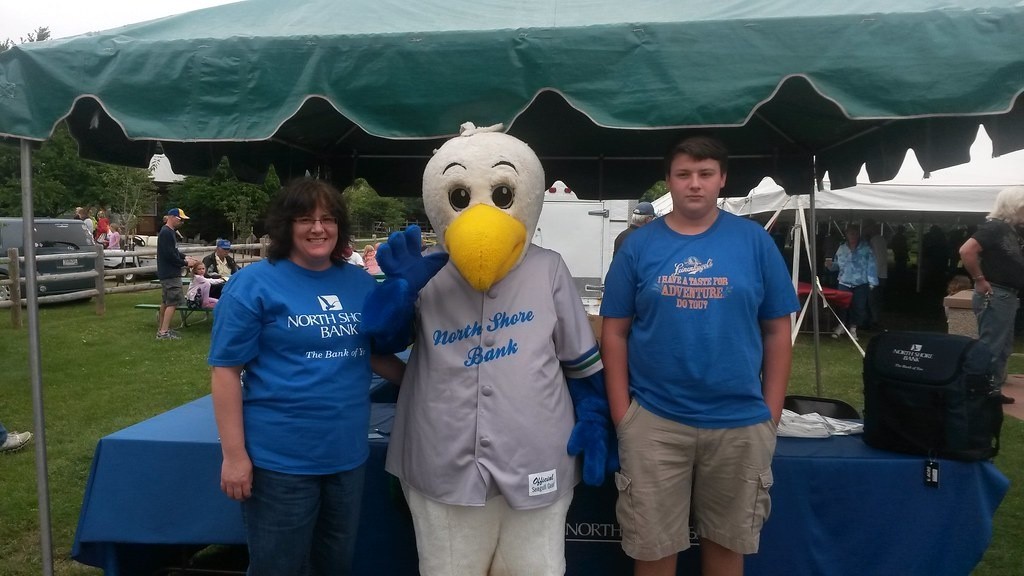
[151,276,229,330]
[797,282,853,342]
[70,349,1010,576]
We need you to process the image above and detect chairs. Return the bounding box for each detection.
[782,396,859,420]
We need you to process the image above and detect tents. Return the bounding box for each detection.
[644,121,1024,351]
[0,1,1024,576]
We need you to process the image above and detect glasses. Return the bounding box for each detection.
[174,216,184,221]
[222,248,229,250]
[293,215,338,227]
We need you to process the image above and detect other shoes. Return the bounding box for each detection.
[831,327,843,339]
[156,328,178,339]
[1002,395,1015,404]
[850,332,858,341]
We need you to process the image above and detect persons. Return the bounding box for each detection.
[861,224,888,329]
[944,275,973,324]
[824,226,880,342]
[185,261,219,309]
[74,205,120,249]
[341,242,385,274]
[919,224,981,283]
[892,225,911,276]
[209,177,382,576]
[613,201,660,262]
[959,186,1024,404]
[599,136,802,576]
[202,239,241,299]
[154,208,196,341]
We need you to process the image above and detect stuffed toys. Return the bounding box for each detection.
[376,121,620,575]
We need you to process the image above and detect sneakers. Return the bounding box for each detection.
[0,431,32,454]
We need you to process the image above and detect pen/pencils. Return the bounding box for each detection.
[374,429,390,436]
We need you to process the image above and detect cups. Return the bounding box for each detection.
[826,258,832,269]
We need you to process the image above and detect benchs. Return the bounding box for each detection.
[135,303,214,312]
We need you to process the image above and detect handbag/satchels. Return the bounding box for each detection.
[862,329,1003,464]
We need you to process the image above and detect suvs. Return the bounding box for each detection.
[0,215,98,308]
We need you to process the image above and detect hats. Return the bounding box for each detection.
[167,208,190,219]
[632,202,657,218]
[216,239,230,249]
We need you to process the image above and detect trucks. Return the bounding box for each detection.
[531,179,639,318]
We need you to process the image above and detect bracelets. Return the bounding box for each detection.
[976,274,984,280]
